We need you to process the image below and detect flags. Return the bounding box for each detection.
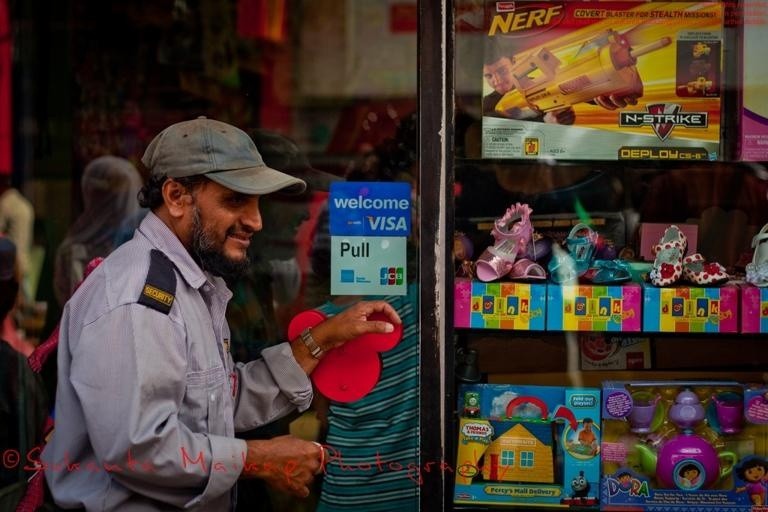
[479,415,560,484]
[609,383,747,489]
[677,39,714,95]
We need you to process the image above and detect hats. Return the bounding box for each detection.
[143,117,307,199]
[246,128,346,193]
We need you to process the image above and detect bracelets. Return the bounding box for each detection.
[300,325,326,363]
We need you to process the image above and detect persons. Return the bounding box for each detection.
[37,113,406,512]
[480,35,575,125]
[577,417,597,448]
[2,128,420,511]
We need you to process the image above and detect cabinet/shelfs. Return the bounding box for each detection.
[417,0,767,511]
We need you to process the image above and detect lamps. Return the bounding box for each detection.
[453,348,483,384]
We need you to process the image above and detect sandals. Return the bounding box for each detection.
[476,202,768,288]
